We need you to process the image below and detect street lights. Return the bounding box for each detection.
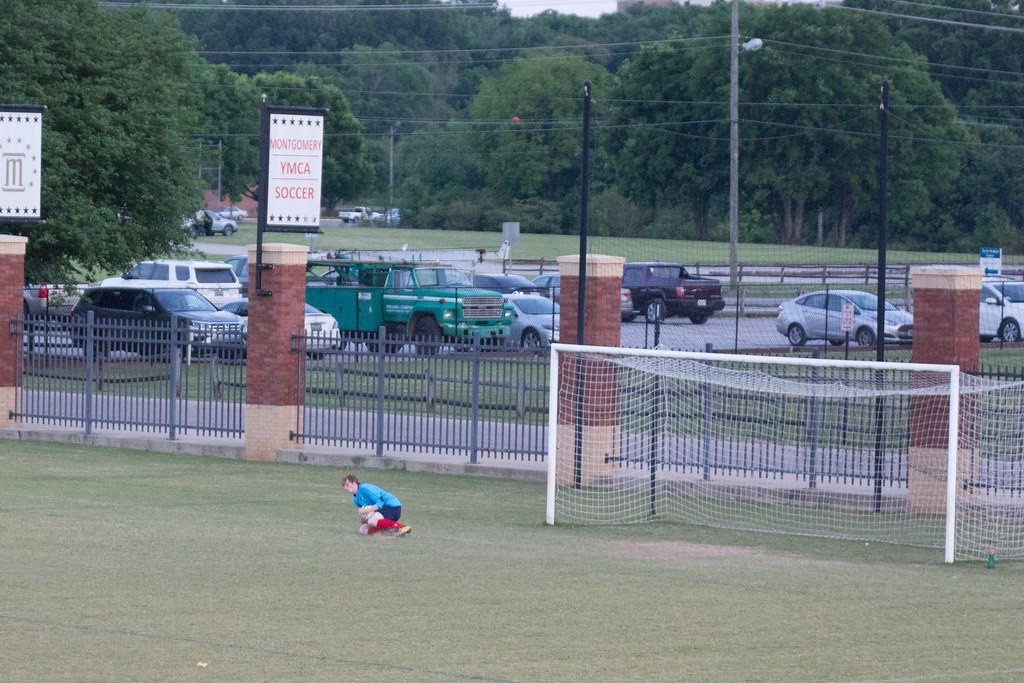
[729,37,765,291]
[388,121,401,205]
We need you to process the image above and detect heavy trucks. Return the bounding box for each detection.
[304,258,515,356]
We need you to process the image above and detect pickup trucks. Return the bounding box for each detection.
[621,262,725,325]
[21,281,90,329]
[338,206,382,224]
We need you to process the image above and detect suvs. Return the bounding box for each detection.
[99,259,242,311]
[69,285,247,365]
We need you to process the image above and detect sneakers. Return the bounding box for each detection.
[397,526,411,537]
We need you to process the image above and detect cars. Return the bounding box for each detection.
[472,273,540,298]
[453,294,561,354]
[776,288,913,348]
[374,208,401,229]
[219,296,341,360]
[225,256,249,298]
[215,206,248,220]
[978,275,1024,343]
[532,273,634,322]
[180,210,238,240]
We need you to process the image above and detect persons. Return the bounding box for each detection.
[341,474,412,536]
[196,209,204,235]
